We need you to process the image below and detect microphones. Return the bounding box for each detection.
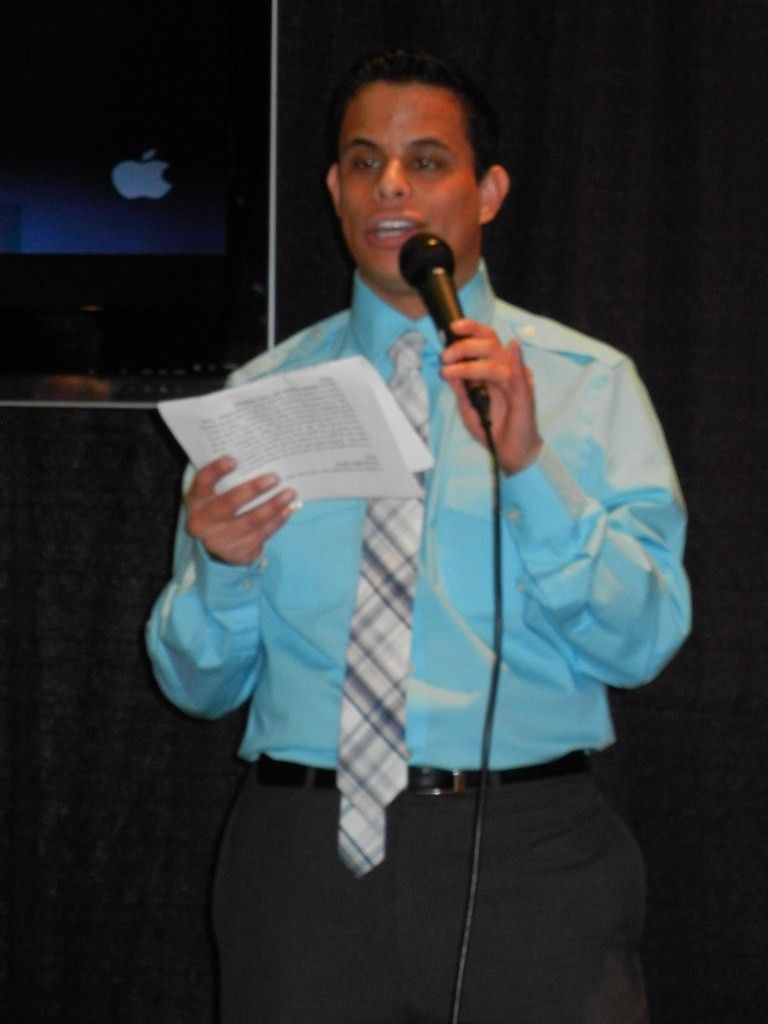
[398,231,493,426]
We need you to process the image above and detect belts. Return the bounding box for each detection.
[245,755,612,795]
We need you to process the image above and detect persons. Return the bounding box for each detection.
[144,53,692,1024]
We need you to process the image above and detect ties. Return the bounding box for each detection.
[335,329,431,879]
